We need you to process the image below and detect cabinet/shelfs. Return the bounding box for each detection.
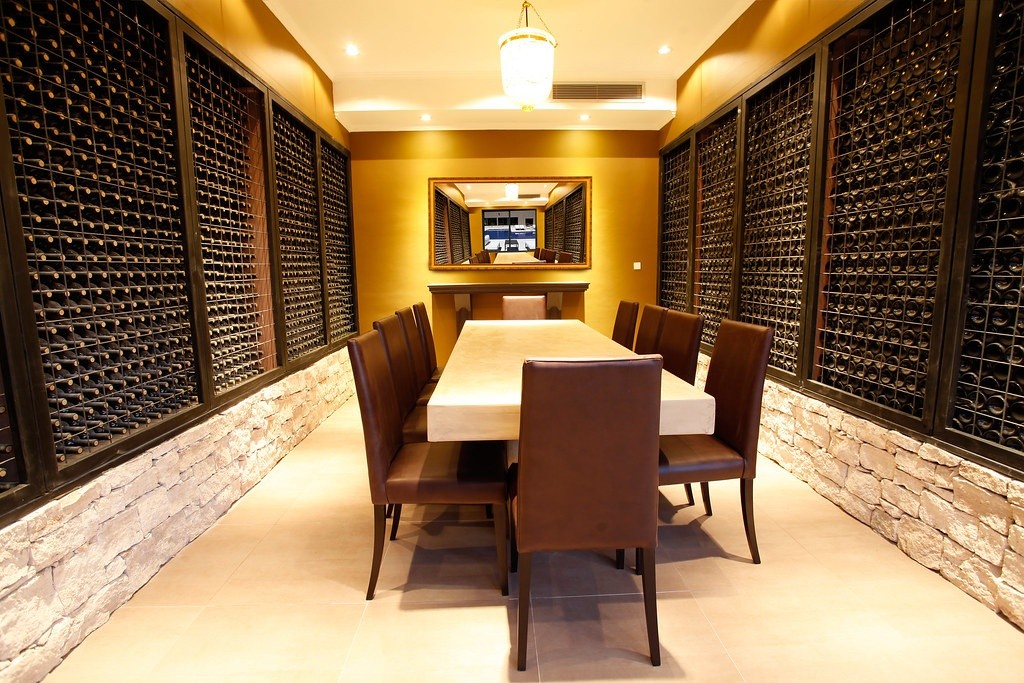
[486,228,536,239]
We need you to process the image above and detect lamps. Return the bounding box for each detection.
[504,182,519,202]
[494,1,559,116]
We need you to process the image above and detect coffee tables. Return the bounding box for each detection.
[505,239,519,250]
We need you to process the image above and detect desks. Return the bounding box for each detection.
[427,283,589,368]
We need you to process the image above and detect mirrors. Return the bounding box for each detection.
[428,176,592,270]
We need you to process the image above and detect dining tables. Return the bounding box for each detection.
[493,252,542,264]
[427,319,716,442]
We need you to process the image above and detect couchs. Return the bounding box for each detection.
[525,240,535,249]
[485,235,500,251]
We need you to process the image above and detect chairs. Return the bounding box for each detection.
[534,248,573,263]
[413,302,445,382]
[347,329,511,601]
[468,250,490,264]
[502,295,546,320]
[508,353,664,672]
[614,318,774,575]
[612,298,640,352]
[655,308,706,388]
[372,314,492,519]
[395,307,439,406]
[634,304,669,355]
